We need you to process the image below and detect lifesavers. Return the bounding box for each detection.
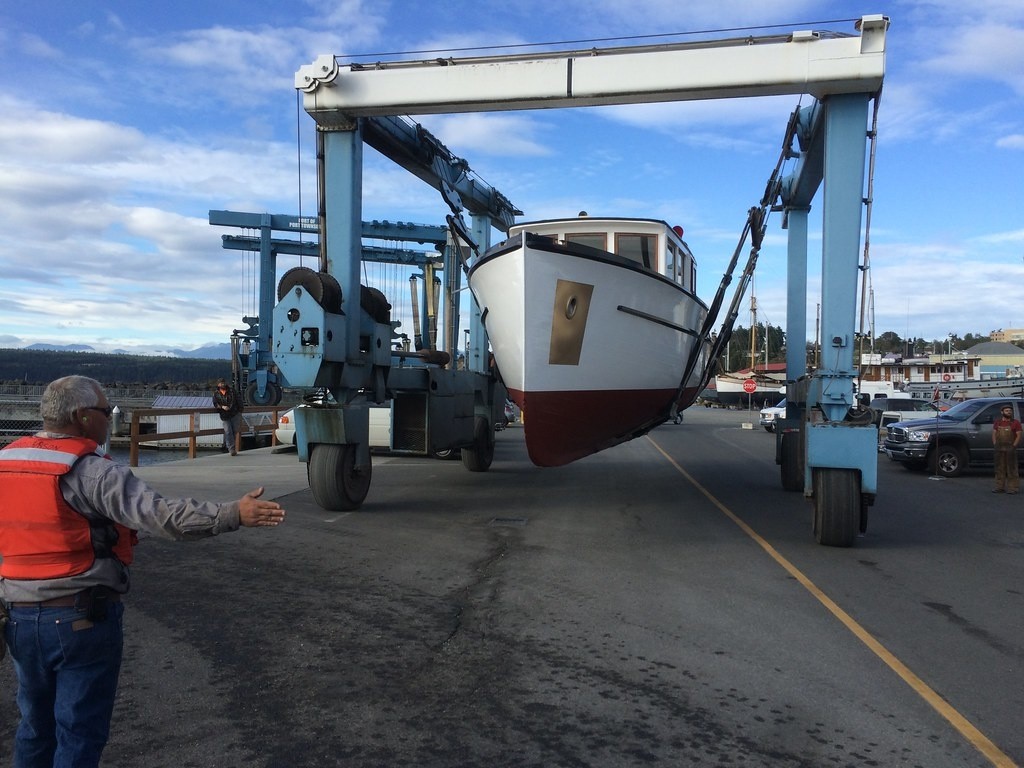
[943,374,950,381]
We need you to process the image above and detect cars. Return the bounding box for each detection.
[759,381,943,453]
[275,388,455,459]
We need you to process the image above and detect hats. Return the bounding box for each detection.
[1000,405,1013,411]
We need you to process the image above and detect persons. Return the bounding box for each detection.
[0,374,287,768]
[991,404,1023,495]
[212,377,244,455]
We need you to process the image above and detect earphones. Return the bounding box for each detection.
[82,416,88,423]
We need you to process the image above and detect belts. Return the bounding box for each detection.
[12,591,121,607]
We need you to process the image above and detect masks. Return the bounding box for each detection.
[219,384,226,388]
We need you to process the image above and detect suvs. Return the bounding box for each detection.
[885,398,1024,477]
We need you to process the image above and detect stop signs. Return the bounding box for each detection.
[743,379,757,393]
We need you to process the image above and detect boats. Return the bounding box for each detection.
[715,375,782,407]
[468,211,714,466]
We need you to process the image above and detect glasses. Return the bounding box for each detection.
[82,405,112,418]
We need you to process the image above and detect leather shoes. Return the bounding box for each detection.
[230,450,237,456]
